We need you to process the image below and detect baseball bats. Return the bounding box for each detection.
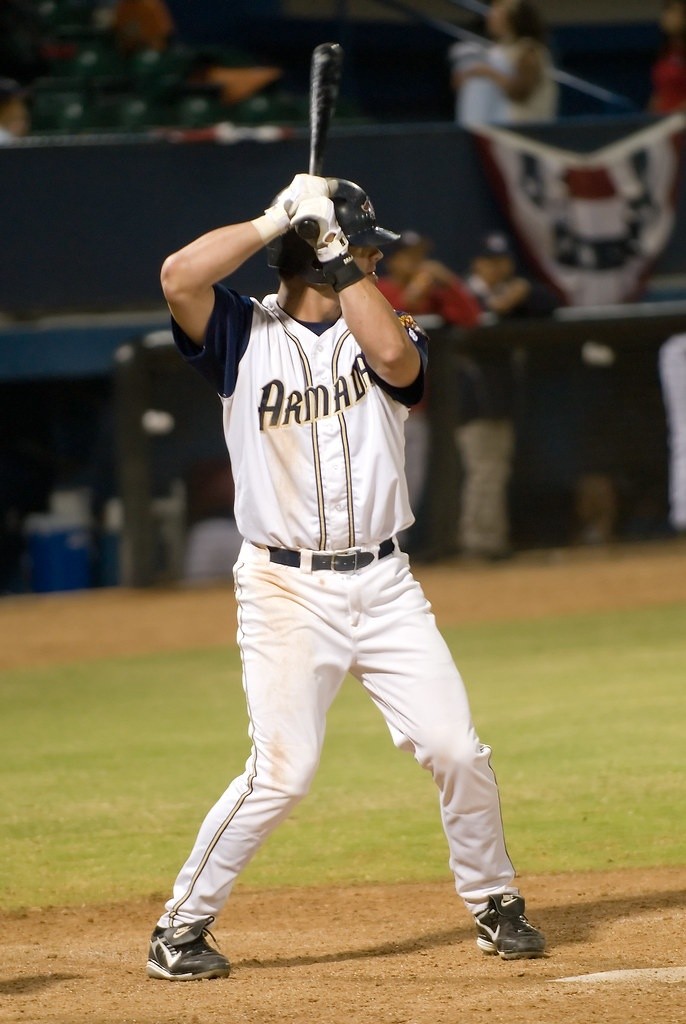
[297,43,344,240]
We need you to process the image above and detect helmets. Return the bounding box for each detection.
[264,177,402,268]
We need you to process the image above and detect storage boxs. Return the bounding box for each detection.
[27,516,119,593]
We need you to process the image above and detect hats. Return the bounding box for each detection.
[473,235,513,259]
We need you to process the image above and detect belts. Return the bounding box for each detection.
[267,537,395,575]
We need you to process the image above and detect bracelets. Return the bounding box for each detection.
[322,252,366,293]
[251,214,281,245]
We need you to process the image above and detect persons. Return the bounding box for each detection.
[0,0,686,571]
[145,174,553,981]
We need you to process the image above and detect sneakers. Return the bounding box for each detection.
[474,893,546,960]
[146,916,231,981]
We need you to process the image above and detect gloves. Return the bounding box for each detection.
[264,173,330,236]
[289,192,350,263]
